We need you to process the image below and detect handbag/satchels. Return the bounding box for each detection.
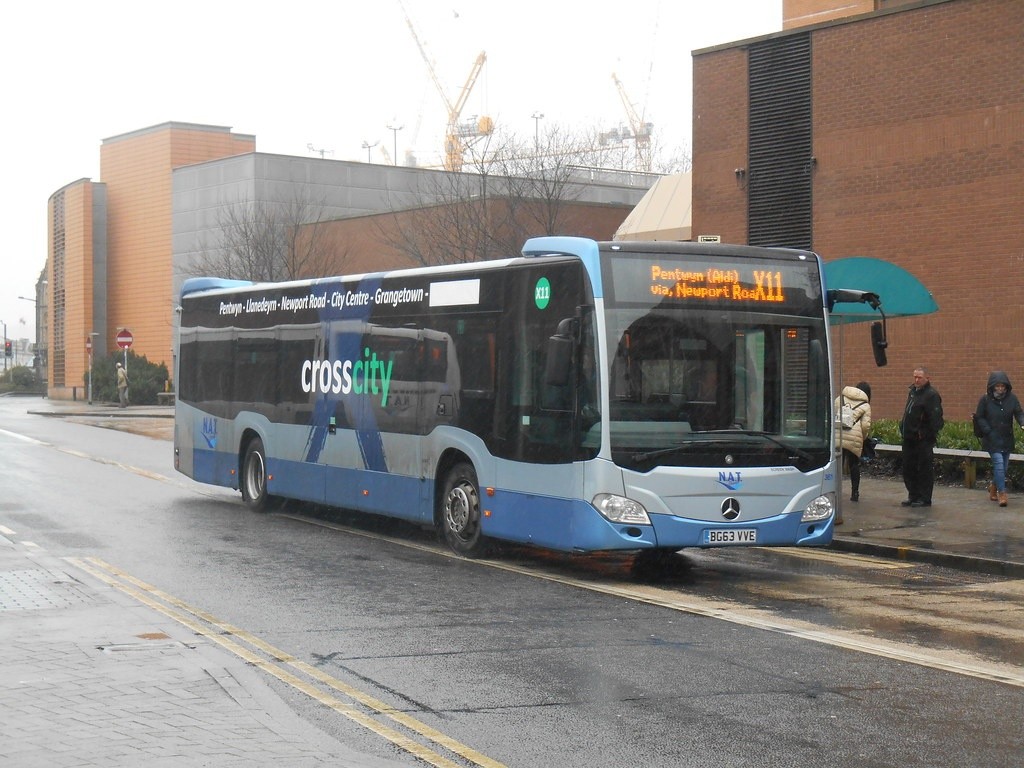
[126,379,130,388]
[973,413,982,438]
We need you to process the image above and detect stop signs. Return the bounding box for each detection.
[86,337,92,354]
[116,331,133,350]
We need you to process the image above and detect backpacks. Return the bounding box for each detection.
[834,395,868,430]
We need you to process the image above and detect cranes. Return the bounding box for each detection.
[611,73,653,171]
[399,2,496,172]
[308,143,333,159]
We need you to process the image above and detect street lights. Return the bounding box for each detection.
[390,125,404,167]
[17,296,40,386]
[362,140,378,164]
[0,320,6,374]
[531,112,545,168]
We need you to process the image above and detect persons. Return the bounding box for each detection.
[898,367,944,507]
[834,381,871,501]
[115,362,130,408]
[975,371,1024,508]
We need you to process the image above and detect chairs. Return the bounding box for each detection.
[678,400,717,430]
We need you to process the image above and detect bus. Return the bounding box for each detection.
[179,319,461,439]
[171,235,889,560]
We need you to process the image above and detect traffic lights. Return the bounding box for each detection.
[4,342,13,357]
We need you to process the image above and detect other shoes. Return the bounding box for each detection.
[849,491,858,501]
[987,484,998,501]
[997,491,1007,506]
[912,499,932,507]
[902,499,912,506]
